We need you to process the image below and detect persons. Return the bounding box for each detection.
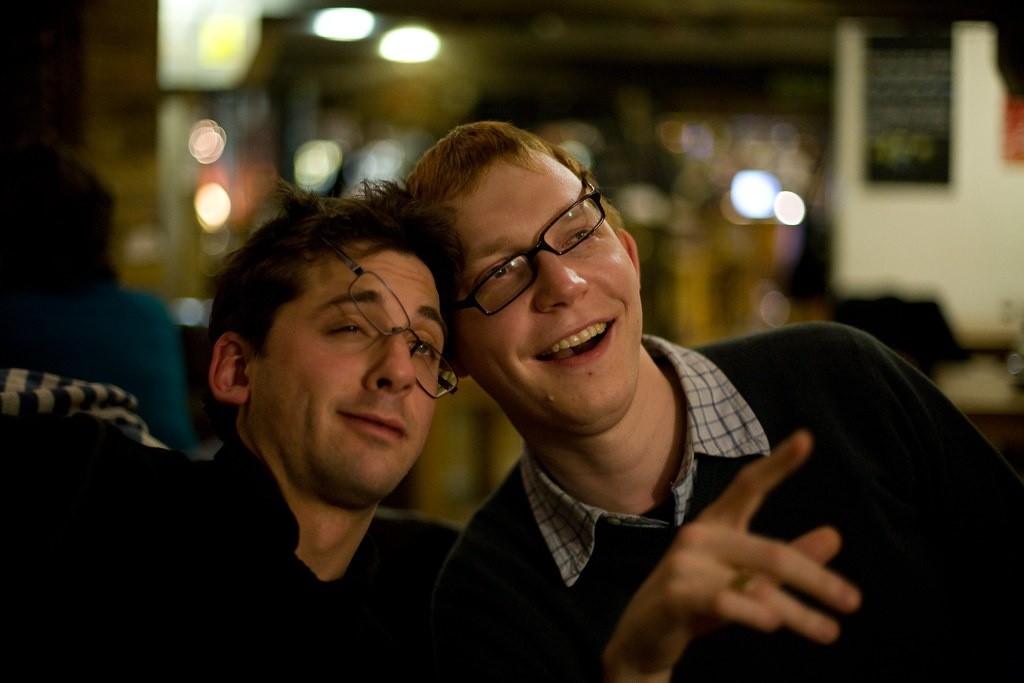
[403,123,1024,683]
[0,150,199,458]
[0,177,470,683]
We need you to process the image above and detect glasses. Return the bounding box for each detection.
[317,232,463,401]
[443,175,608,317]
